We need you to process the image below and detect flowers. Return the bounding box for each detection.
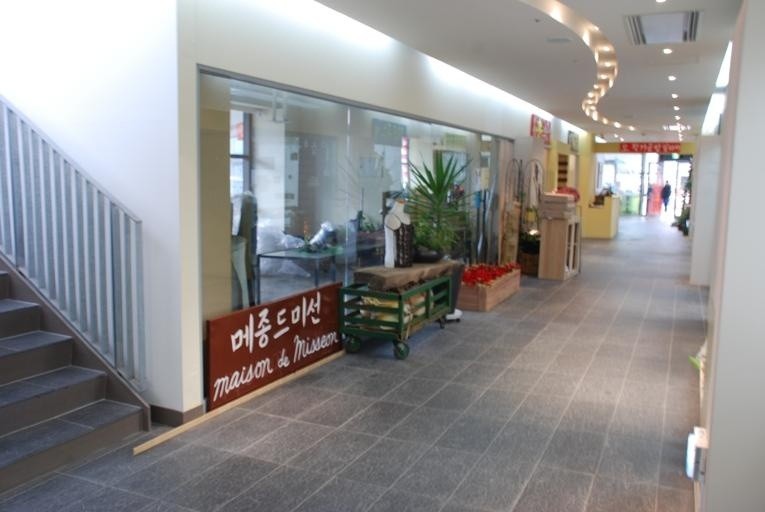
[461,262,521,288]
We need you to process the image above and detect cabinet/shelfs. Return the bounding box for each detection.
[538,216,582,280]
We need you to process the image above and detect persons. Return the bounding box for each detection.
[379,199,418,271]
[660,180,672,212]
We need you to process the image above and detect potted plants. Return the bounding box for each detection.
[404,148,477,263]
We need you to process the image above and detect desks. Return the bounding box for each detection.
[256,241,385,306]
